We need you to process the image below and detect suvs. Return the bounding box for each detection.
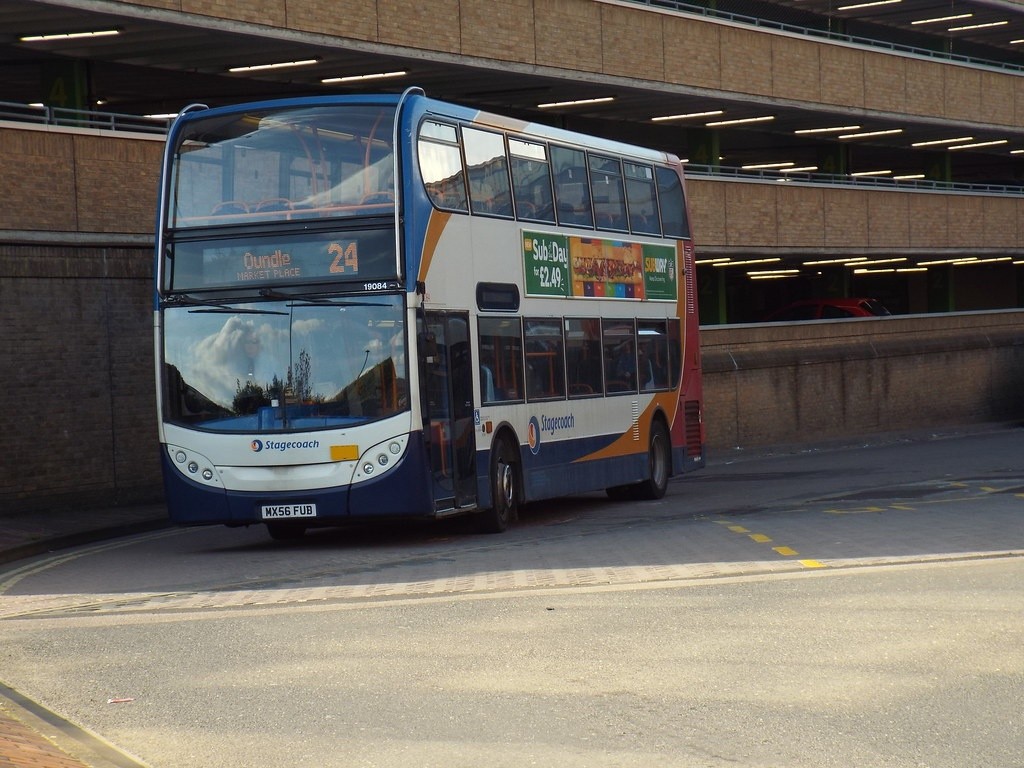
[760,298,892,323]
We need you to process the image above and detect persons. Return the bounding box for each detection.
[227,327,287,387]
[539,183,575,223]
[616,340,654,390]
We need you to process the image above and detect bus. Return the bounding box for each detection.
[153,86,706,542]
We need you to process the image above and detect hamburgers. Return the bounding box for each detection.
[572,247,643,284]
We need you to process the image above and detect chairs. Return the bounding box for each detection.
[211,187,654,233]
[570,379,631,397]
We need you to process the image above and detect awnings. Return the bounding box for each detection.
[523,324,660,352]
